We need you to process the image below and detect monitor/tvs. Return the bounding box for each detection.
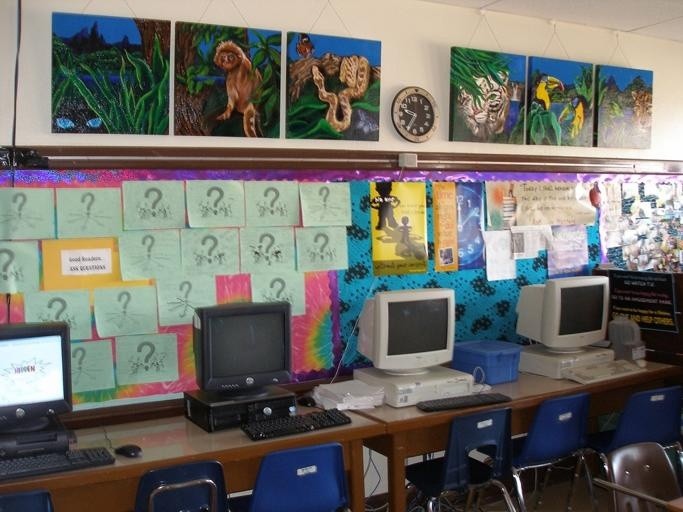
[193,300,292,397]
[515,276,610,354]
[0,322,71,431]
[356,286,453,378]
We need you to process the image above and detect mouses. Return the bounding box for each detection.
[117,443,143,458]
[472,383,492,392]
[297,397,317,407]
[634,359,648,368]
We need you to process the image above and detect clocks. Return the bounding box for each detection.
[391,86,439,143]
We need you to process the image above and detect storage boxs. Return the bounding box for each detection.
[452,340,524,386]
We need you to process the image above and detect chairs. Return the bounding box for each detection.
[135,459,227,512]
[594,442,683,512]
[0,488,53,512]
[405,407,516,512]
[510,392,599,512]
[248,442,349,512]
[567,387,683,512]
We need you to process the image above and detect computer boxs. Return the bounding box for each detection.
[183,384,297,433]
[0,416,70,460]
[518,344,614,380]
[353,365,475,408]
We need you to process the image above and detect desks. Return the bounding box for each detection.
[0,405,387,512]
[348,360,680,512]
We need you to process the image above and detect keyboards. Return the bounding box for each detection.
[240,407,348,440]
[564,358,647,385]
[420,390,511,414]
[0,445,116,477]
